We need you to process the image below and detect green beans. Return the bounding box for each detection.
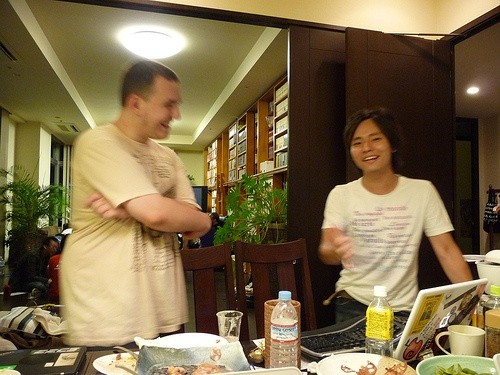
[435,363,494,375]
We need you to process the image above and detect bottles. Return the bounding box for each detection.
[269,290,299,367]
[364,285,394,358]
[470,284,500,330]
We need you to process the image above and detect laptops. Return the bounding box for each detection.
[390,277,492,363]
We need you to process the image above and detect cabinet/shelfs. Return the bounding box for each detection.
[204,77,288,226]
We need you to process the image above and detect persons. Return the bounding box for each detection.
[58,60,212,351]
[318,110,473,326]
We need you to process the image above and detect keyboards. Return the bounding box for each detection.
[298,314,411,362]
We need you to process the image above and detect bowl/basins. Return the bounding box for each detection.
[475,260,500,293]
[135,331,229,350]
[416,355,497,375]
[138,340,251,375]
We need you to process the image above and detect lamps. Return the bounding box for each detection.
[122,31,177,59]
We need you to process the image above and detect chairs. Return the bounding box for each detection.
[181,238,317,341]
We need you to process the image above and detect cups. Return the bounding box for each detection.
[435,324,486,356]
[216,310,243,343]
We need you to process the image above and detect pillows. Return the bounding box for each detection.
[0,305,51,340]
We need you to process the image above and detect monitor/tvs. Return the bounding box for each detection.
[193,186,209,214]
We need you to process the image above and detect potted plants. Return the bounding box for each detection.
[0,165,68,293]
[214,173,288,307]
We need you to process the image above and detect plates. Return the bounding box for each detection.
[92,350,139,375]
[316,353,416,375]
